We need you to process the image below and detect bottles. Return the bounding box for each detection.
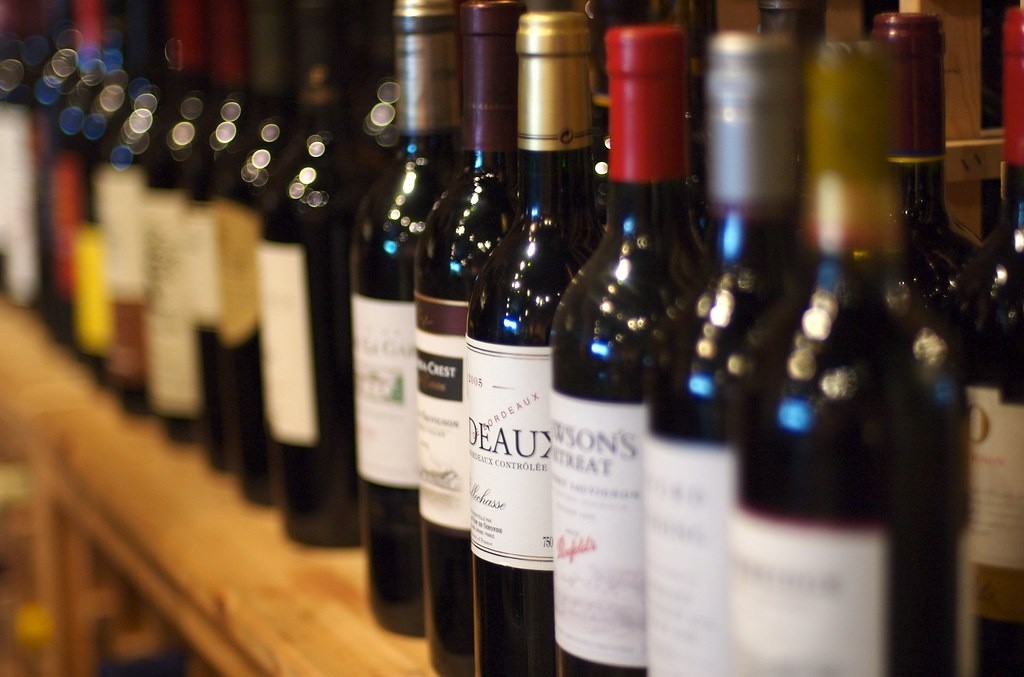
[0,0,1024,677]
